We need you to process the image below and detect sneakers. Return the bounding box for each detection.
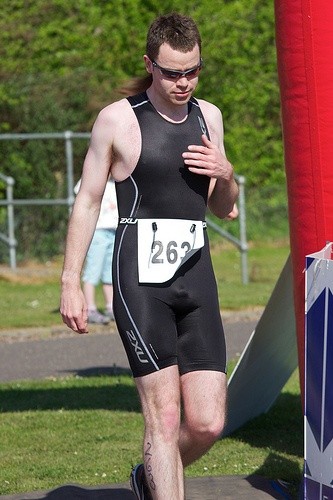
[87,312,110,324]
[104,310,115,321]
[129,463,152,500]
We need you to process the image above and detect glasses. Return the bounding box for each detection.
[149,55,202,80]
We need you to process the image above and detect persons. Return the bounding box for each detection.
[59,12,238,500]
[74,173,119,325]
